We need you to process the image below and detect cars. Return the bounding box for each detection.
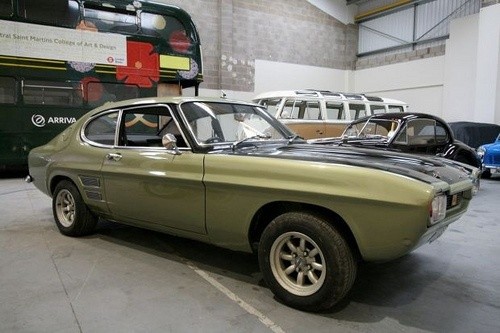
[416,120,499,151]
[306,110,482,182]
[28,92,482,309]
[477,127,500,176]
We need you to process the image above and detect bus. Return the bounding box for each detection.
[1,0,204,171]
[238,89,408,140]
